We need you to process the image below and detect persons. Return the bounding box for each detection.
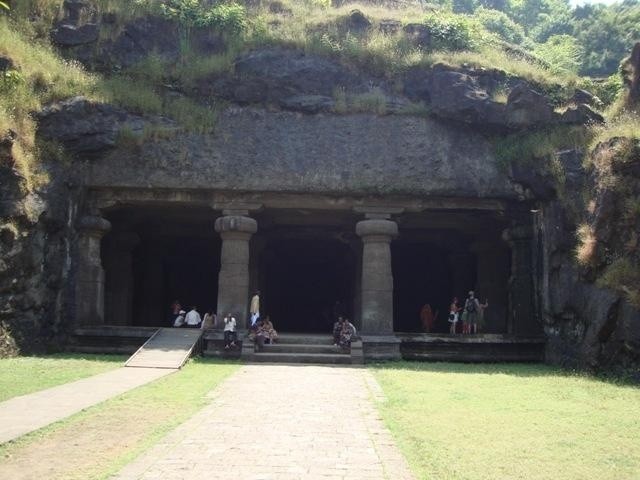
[332,317,357,350]
[248,289,278,344]
[171,299,181,315]
[448,290,490,334]
[185,306,201,326]
[224,312,237,351]
[421,303,434,330]
[173,310,189,327]
[200,306,218,330]
